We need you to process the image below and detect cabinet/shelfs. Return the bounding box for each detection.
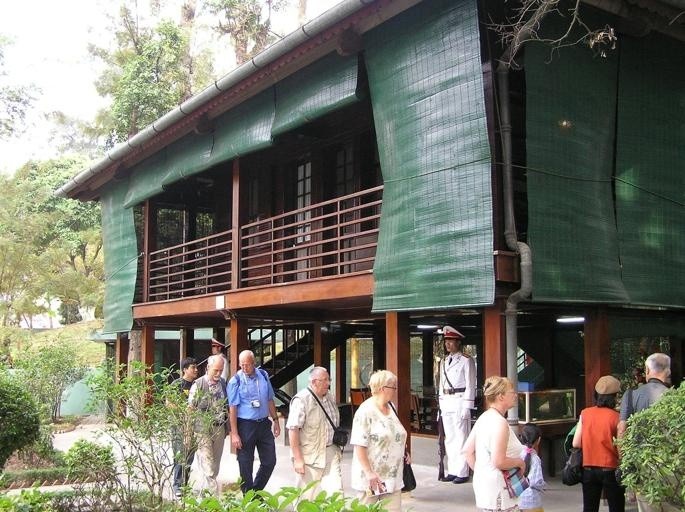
[518,388,577,424]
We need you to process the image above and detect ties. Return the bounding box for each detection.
[449,357,452,365]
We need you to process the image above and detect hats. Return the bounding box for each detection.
[595,376,621,395]
[442,325,465,341]
[211,337,225,349]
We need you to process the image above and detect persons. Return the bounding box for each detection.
[571,375,626,511]
[182,354,232,493]
[438,325,477,484]
[615,352,679,511]
[462,375,526,512]
[349,370,412,512]
[517,422,548,512]
[285,366,346,510]
[210,337,230,383]
[165,357,199,504]
[226,350,281,498]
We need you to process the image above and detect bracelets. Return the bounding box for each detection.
[273,417,278,421]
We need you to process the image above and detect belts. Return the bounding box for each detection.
[443,388,466,394]
[253,419,267,423]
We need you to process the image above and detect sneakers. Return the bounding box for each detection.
[440,474,469,483]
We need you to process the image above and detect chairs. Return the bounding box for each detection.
[349,386,439,435]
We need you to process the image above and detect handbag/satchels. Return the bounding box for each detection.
[402,463,416,492]
[562,448,583,486]
